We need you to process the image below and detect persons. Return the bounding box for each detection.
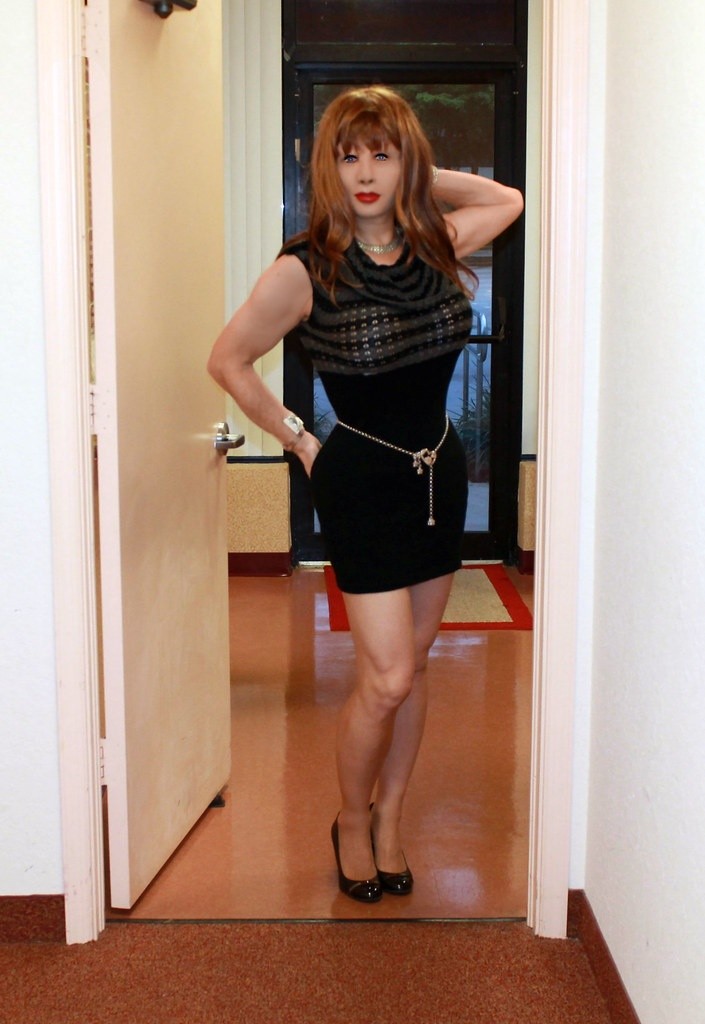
[209,85,526,904]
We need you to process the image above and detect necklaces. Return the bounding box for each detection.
[350,222,405,255]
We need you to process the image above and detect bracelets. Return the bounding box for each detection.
[275,427,306,452]
[426,163,440,196]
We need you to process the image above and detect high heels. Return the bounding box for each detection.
[330,811,382,902]
[367,802,413,893]
